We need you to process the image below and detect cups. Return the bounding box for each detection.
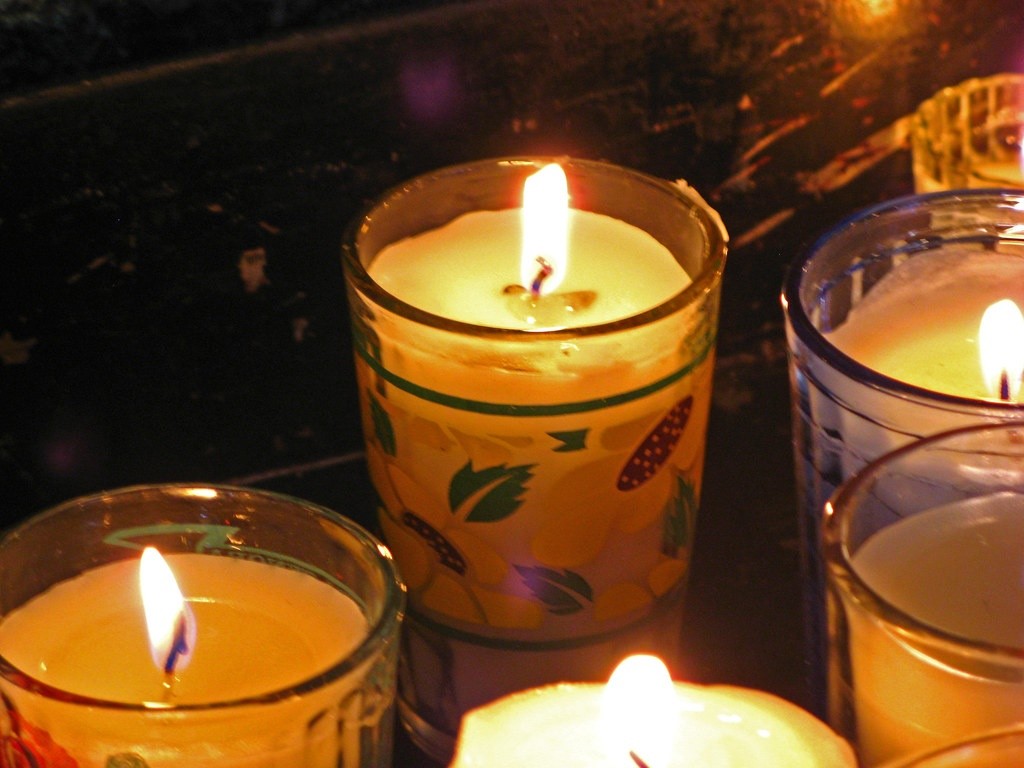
[0,483,408,768]
[907,73,1024,195]
[336,155,730,768]
[447,682,856,768]
[817,418,1024,766]
[782,187,1024,727]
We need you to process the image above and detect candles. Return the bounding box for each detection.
[0,482,401,768]
[787,186,1024,614]
[451,653,855,768]
[346,157,730,756]
